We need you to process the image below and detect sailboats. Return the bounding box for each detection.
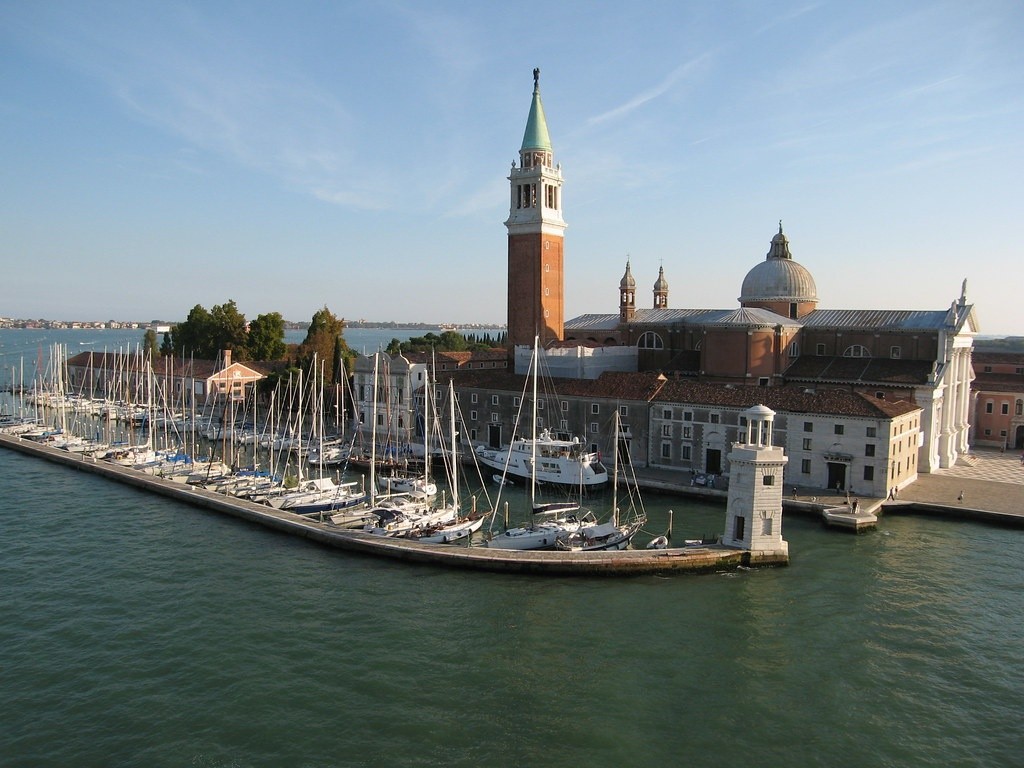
[0,332,677,549]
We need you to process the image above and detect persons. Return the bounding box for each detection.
[886,487,895,501]
[792,487,798,500]
[1021,452,1024,465]
[690,468,696,479]
[1000,445,1004,455]
[895,486,898,497]
[851,498,860,514]
[836,481,840,493]
[958,491,964,504]
